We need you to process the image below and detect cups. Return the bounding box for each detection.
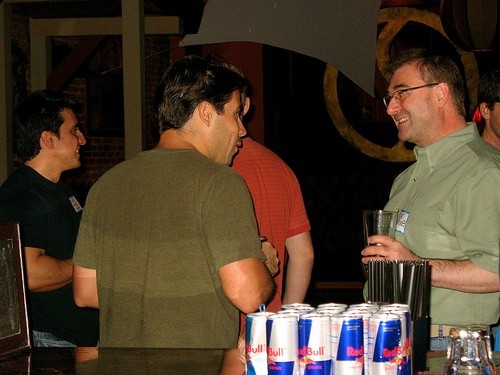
[445,324,499,374]
[363,209,399,276]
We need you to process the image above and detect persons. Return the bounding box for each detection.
[224,68,316,351]
[361,44,500,352]
[476,60,500,352]
[0,87,101,348]
[70,54,280,349]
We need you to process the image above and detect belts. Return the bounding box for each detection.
[430,323,470,337]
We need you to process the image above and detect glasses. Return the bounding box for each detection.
[383,82,441,108]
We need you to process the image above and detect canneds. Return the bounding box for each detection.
[245,302,413,375]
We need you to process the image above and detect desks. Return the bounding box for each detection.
[0,346,226,375]
